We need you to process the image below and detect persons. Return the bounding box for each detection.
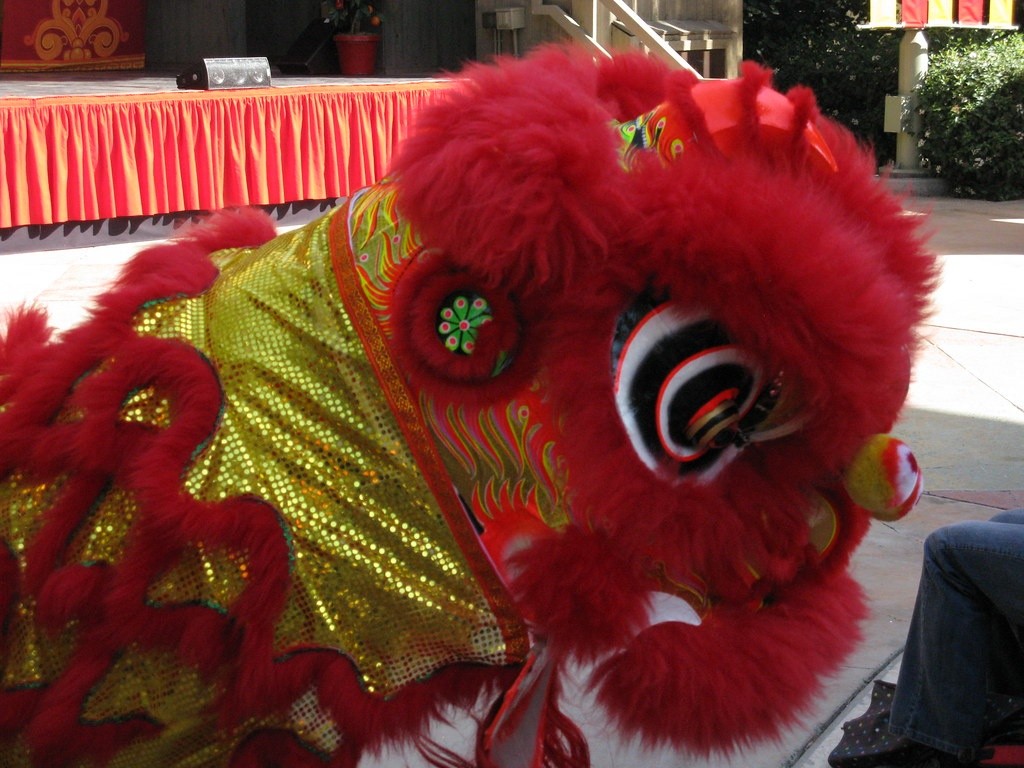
[888,509,1024,768]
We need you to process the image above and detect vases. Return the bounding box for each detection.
[334,33,380,77]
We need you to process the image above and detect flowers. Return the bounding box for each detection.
[322,0,383,34]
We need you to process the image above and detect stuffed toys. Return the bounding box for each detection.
[0,43,941,768]
